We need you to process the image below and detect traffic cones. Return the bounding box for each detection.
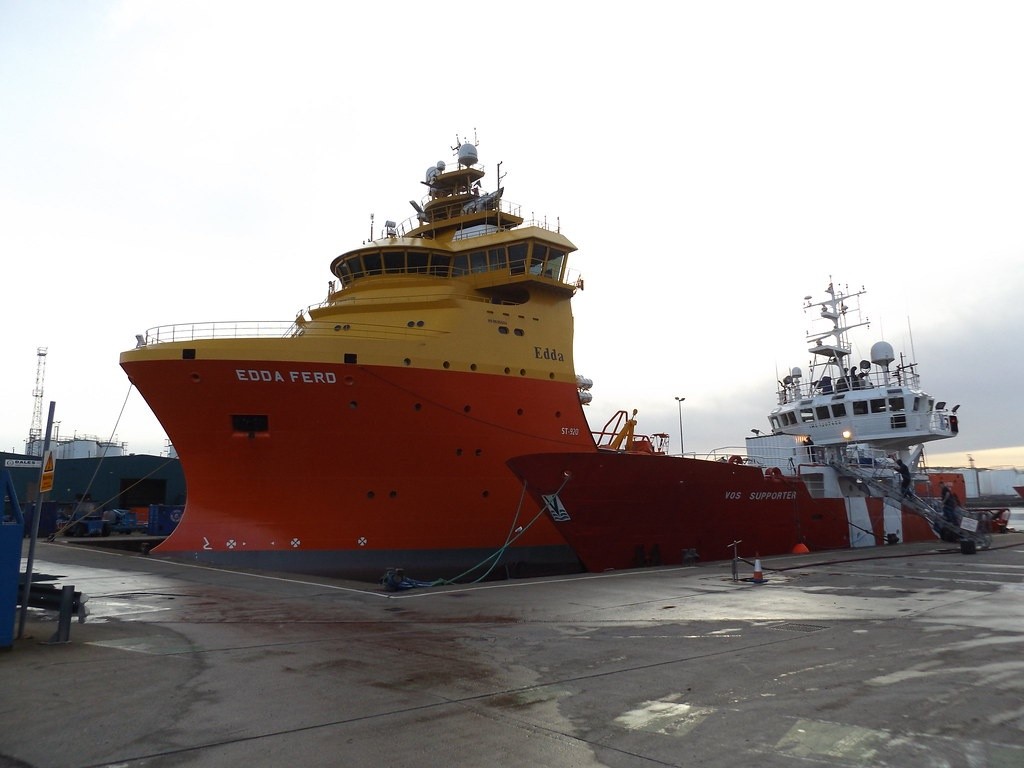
[750,552,768,582]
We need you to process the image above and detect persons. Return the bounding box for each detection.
[893,459,914,501]
[938,481,954,528]
[802,436,816,462]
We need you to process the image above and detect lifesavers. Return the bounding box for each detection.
[729,455,743,465]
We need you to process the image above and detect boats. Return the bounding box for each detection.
[505,274,1010,575]
[120,127,673,584]
